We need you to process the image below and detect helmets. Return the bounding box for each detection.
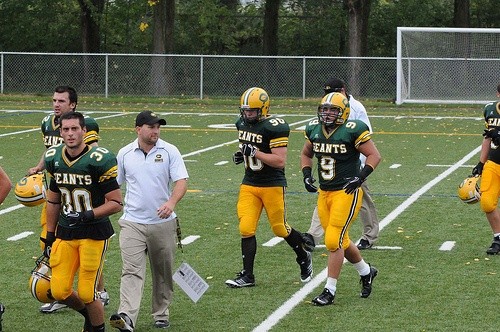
[457,174,482,204]
[15,171,48,207]
[30,261,57,303]
[239,87,270,117]
[318,92,350,125]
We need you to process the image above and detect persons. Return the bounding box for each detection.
[224,87,313,288]
[470,85,500,254]
[0,85,188,332]
[300,93,382,304]
[297,78,380,249]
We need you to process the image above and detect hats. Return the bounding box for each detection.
[136,111,166,126]
[323,78,344,90]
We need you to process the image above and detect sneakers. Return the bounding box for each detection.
[0,303,5,331]
[95,288,109,306]
[39,300,68,314]
[110,313,134,332]
[297,231,315,252]
[486,233,500,254]
[313,288,336,305]
[358,262,378,298]
[224,269,255,287]
[155,320,169,327]
[296,246,313,282]
[356,239,370,248]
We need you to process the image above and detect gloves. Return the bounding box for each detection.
[342,174,364,194]
[62,211,87,227]
[241,141,259,158]
[233,151,244,164]
[483,129,499,141]
[303,174,317,193]
[472,161,484,175]
[43,236,55,258]
